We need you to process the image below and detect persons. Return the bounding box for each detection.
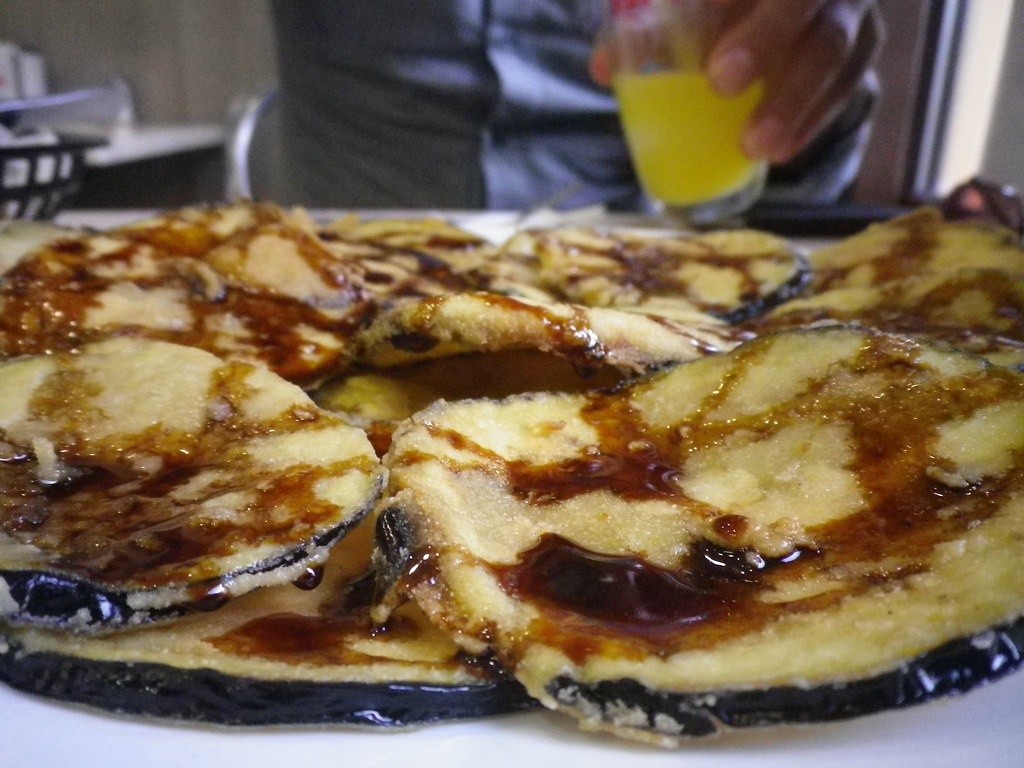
[272,0,887,221]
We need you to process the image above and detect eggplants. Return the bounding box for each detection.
[1,193,1020,744]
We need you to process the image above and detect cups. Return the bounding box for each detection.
[610,0,767,225]
